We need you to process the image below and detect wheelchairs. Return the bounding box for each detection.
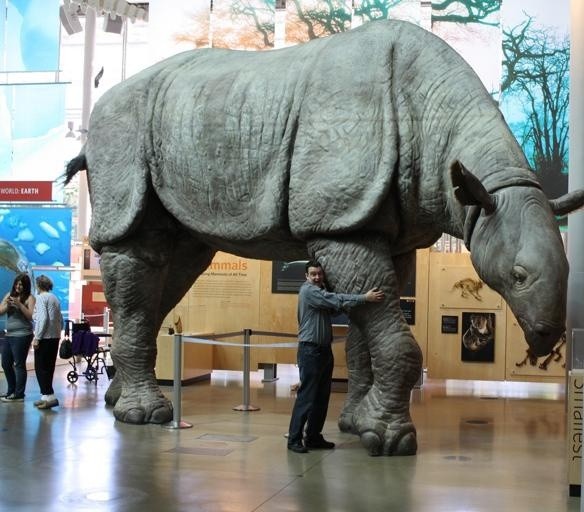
[60,318,114,383]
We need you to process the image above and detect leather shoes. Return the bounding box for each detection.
[288,444,308,452]
[306,440,334,448]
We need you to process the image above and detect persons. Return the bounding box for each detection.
[288,261,385,453]
[0,274,36,402]
[32,275,64,409]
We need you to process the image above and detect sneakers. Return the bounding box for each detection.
[0,393,24,402]
[34,399,59,409]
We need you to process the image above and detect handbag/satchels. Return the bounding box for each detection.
[59,339,73,359]
